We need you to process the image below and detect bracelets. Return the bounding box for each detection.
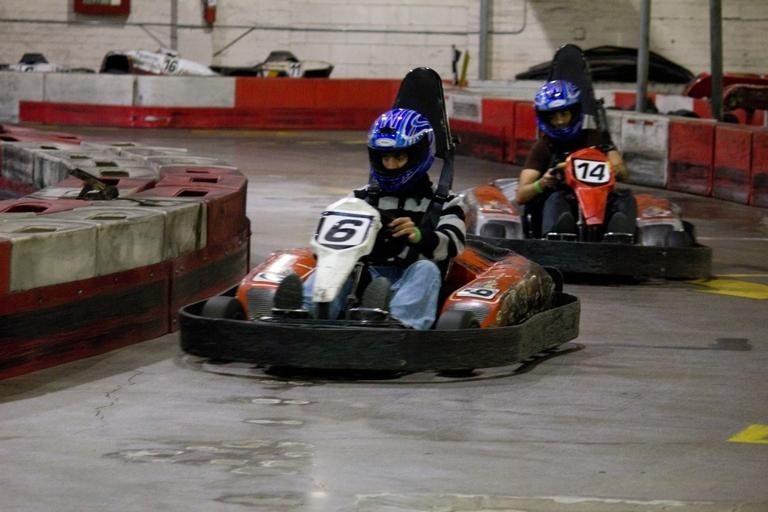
[409,227,422,244]
[534,180,543,193]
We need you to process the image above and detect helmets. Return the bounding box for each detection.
[365,108,437,188]
[533,80,583,138]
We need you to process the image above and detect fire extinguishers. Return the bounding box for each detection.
[205,0,217,24]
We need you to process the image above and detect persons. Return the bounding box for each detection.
[516,80,638,233]
[273,108,469,330]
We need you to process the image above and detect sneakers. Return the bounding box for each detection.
[362,276,391,320]
[608,212,631,232]
[556,212,575,232]
[274,274,303,309]
